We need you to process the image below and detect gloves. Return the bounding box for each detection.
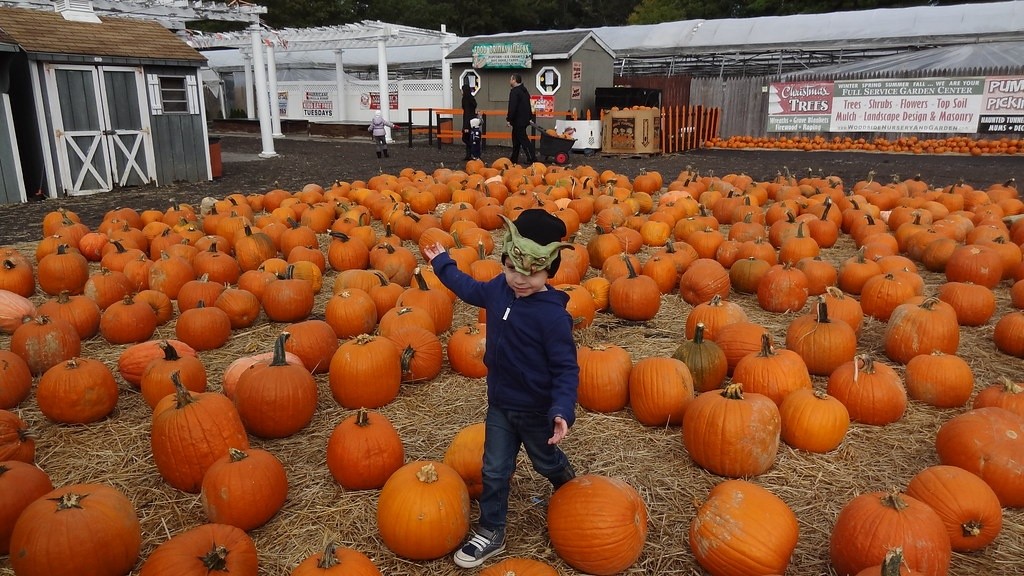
[394,125,400,129]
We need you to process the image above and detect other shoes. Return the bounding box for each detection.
[524,157,537,165]
[509,158,518,164]
[463,156,472,161]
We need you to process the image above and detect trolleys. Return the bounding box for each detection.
[528,121,578,165]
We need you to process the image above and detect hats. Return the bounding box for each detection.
[374,110,382,118]
[501,209,568,279]
[470,118,481,128]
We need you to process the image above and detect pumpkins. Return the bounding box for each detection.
[611,105,658,111]
[703,136,1024,155]
[0,159,1024,576]
[546,129,572,140]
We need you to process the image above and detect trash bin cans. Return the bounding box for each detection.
[207,135,223,179]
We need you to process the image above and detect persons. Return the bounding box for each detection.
[368,111,402,158]
[466,118,482,161]
[425,209,575,569]
[462,84,477,159]
[506,73,538,165]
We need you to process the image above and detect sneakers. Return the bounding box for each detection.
[453,527,506,569]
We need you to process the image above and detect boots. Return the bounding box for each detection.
[376,151,382,159]
[383,149,389,158]
